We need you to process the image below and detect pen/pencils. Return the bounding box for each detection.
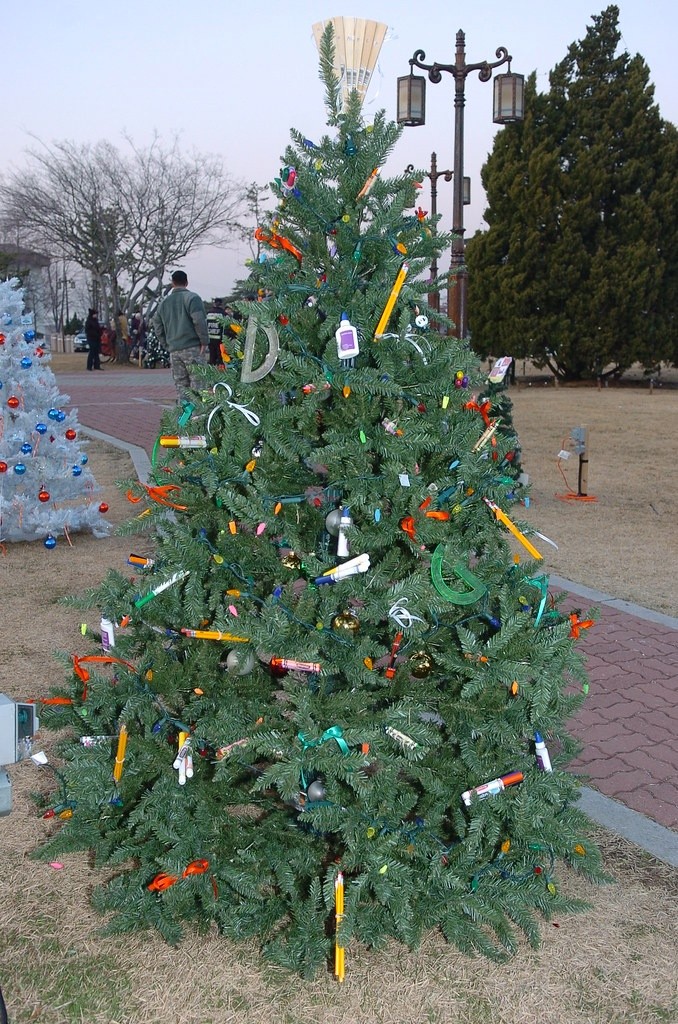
[47,127,552,985]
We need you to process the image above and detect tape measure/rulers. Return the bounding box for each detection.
[239,306,279,385]
[429,543,489,606]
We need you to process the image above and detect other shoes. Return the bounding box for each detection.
[87,368,92,371]
[94,368,104,370]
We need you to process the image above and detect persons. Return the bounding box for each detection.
[85,308,106,371]
[206,293,255,365]
[101,309,146,360]
[152,270,211,408]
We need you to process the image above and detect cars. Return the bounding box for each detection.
[73,332,90,353]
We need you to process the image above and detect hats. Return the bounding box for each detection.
[89,308,97,315]
[214,298,223,303]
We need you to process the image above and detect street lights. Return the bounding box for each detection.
[402,150,470,331]
[396,27,527,344]
